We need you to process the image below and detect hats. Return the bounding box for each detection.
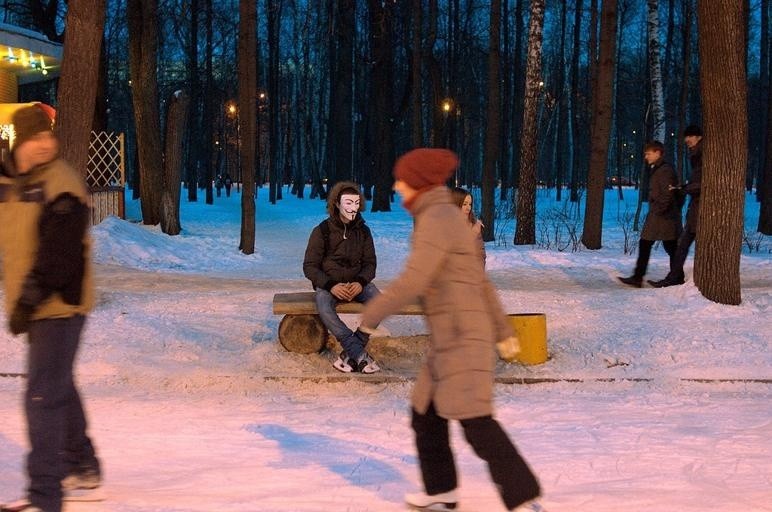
[9,105,52,153]
[393,149,458,188]
[683,126,702,136]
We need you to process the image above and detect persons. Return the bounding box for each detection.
[618,140,683,288]
[361,148,548,512]
[659,125,702,288]
[0,102,107,512]
[303,182,381,374]
[452,186,486,269]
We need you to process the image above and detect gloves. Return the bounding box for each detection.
[10,304,31,335]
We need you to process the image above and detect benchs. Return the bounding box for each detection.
[274,293,425,354]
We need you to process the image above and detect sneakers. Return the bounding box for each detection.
[60,477,102,492]
[334,352,380,374]
[407,490,459,510]
[1,497,40,512]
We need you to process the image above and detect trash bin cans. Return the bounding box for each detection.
[504,313,549,366]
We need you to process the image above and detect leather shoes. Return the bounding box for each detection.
[619,277,642,287]
[648,278,669,288]
